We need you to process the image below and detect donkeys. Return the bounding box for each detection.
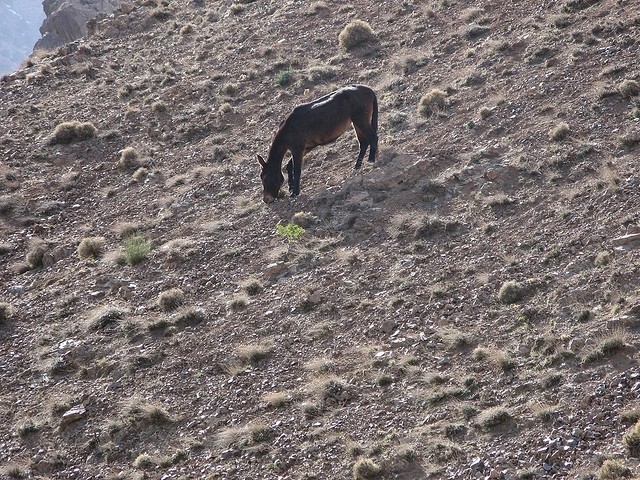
[255,84,379,203]
